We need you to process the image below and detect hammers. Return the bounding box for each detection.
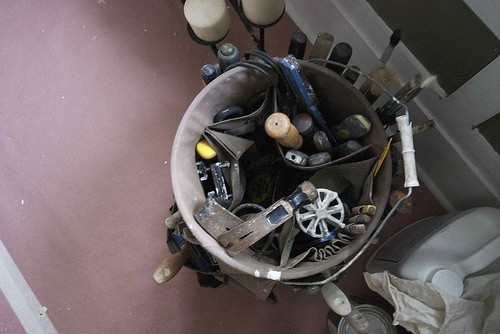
[215,180,320,255]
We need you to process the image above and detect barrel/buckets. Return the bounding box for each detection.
[170,58,393,283]
